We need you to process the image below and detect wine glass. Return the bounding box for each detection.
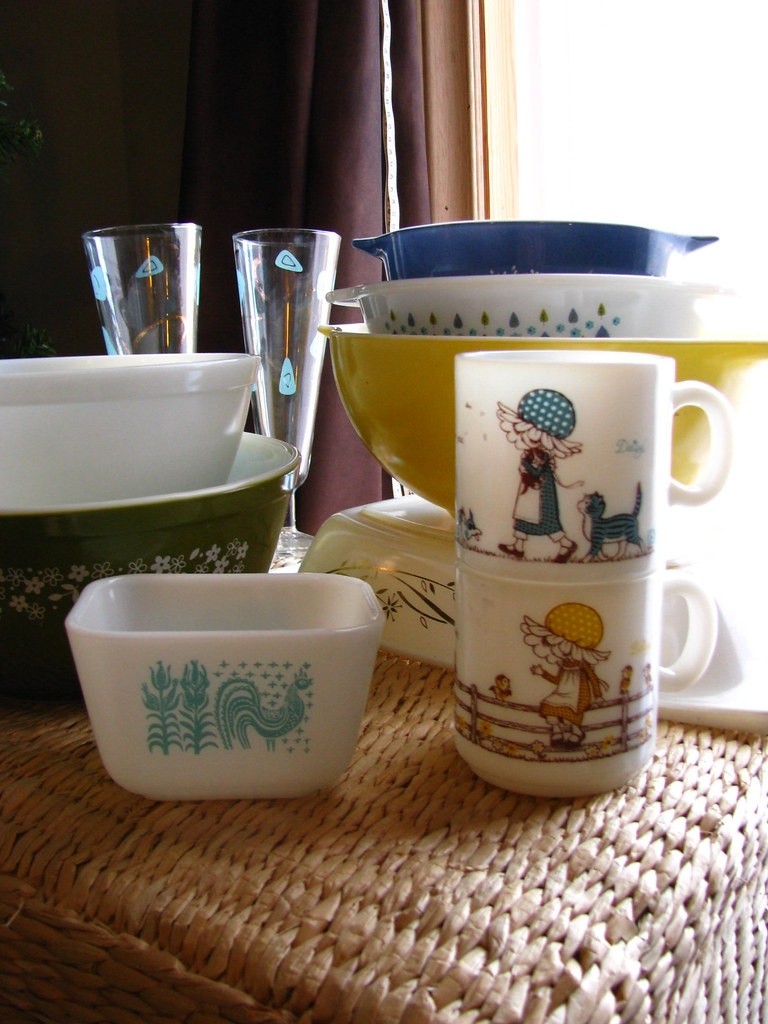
[232,228,342,571]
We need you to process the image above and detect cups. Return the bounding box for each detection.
[453,557,718,798]
[448,349,741,585]
[83,223,203,356]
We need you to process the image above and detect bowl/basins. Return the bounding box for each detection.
[317,322,768,522]
[296,493,767,730]
[0,432,301,692]
[351,221,718,283]
[64,574,386,801]
[1,353,260,512]
